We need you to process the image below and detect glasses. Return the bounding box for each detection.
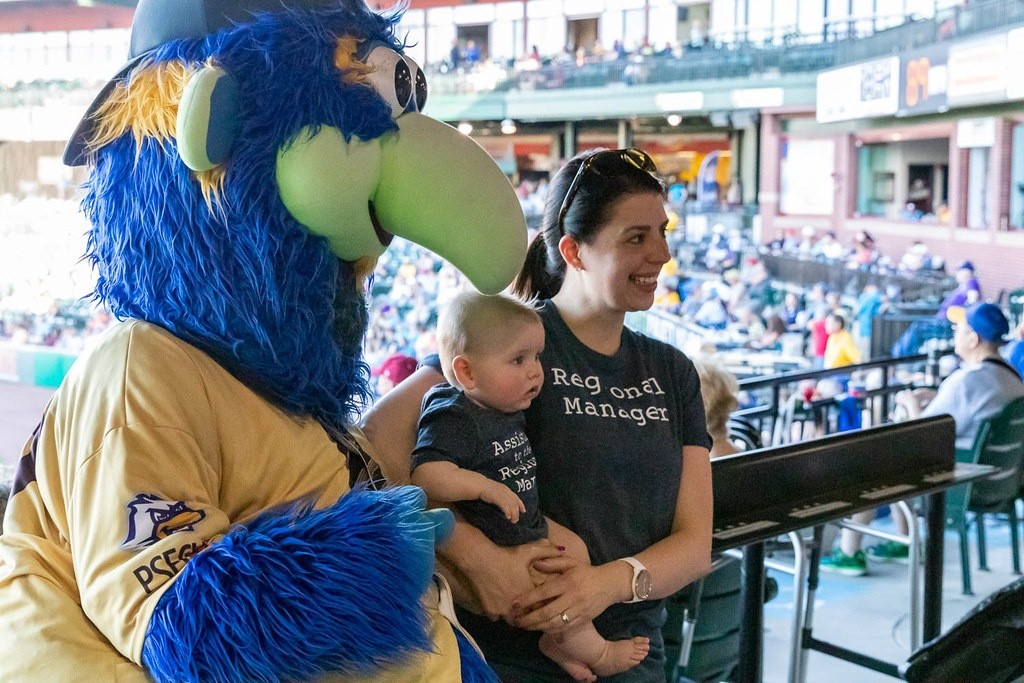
[558,148,657,233]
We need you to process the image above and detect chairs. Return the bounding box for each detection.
[666,543,745,682]
[915,393,1024,595]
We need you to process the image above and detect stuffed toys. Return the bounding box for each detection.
[0,0,496,683]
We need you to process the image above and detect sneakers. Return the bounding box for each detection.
[866,540,923,564]
[818,545,868,576]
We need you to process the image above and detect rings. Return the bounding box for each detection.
[561,612,569,624]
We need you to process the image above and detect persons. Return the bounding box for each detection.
[817,304,1024,576]
[361,147,715,683]
[691,360,744,461]
[2,31,1024,425]
[411,291,651,683]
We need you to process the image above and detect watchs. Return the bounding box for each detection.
[618,557,652,604]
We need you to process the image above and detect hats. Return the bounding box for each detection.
[959,261,974,272]
[945,303,1012,345]
